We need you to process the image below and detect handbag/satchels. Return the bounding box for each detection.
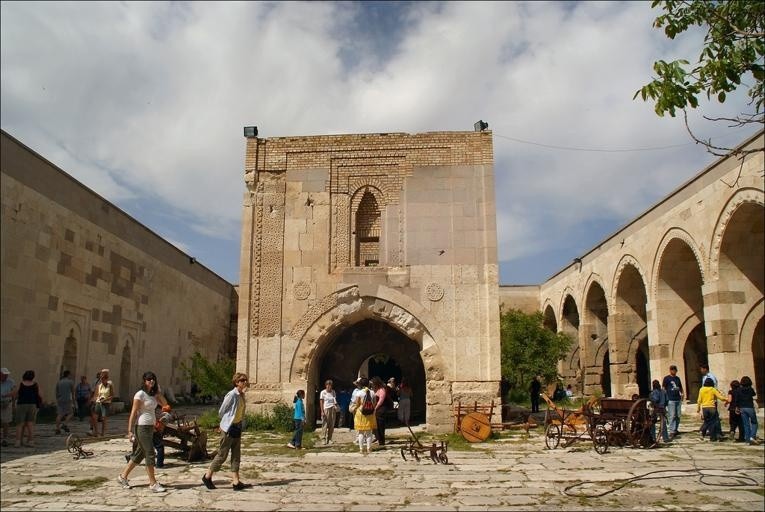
[375,394,393,418]
[334,403,341,412]
[228,426,241,438]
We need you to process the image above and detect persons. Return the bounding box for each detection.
[529,376,541,412]
[124,383,171,468]
[119,371,167,492]
[319,376,414,454]
[287,390,307,450]
[501,376,512,404]
[201,372,253,490]
[648,363,760,449]
[0,367,117,449]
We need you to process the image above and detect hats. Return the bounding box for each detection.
[352,378,362,384]
[0,367,11,376]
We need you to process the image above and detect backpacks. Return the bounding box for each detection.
[359,391,375,417]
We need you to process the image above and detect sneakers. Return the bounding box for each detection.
[0,437,33,447]
[148,483,168,494]
[116,473,133,491]
[359,447,375,454]
[286,442,306,450]
[200,472,218,491]
[231,480,254,492]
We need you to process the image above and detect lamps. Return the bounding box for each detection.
[472,119,490,134]
[243,125,258,139]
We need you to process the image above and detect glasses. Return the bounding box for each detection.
[237,378,247,383]
[145,378,155,381]
